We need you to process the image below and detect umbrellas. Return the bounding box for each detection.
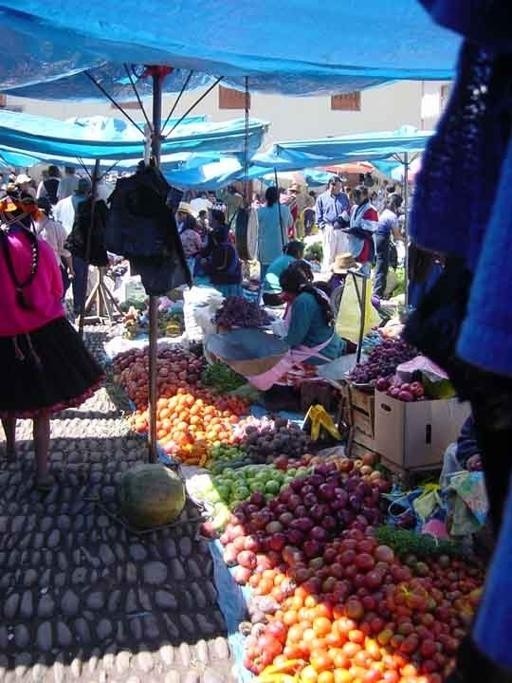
[0,0,461,462]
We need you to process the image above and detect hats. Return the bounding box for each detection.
[13,174,32,186]
[176,201,192,213]
[329,250,363,277]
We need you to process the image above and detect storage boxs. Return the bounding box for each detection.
[373,386,471,468]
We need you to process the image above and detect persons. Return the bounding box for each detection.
[172,171,421,410]
[1,166,112,486]
[437,410,483,485]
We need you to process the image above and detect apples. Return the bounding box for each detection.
[113,336,482,683]
